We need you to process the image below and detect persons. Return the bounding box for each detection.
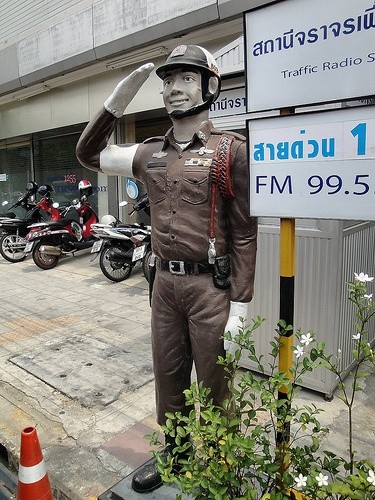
[76,45,258,493]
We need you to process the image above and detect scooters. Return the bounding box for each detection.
[0,184,84,264]
[88,192,151,283]
[21,178,102,270]
[0,179,46,241]
[128,223,152,282]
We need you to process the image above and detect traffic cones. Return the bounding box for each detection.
[12,425,56,500]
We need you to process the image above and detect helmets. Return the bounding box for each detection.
[101,215,116,225]
[37,184,54,196]
[77,179,93,190]
[26,182,38,192]
